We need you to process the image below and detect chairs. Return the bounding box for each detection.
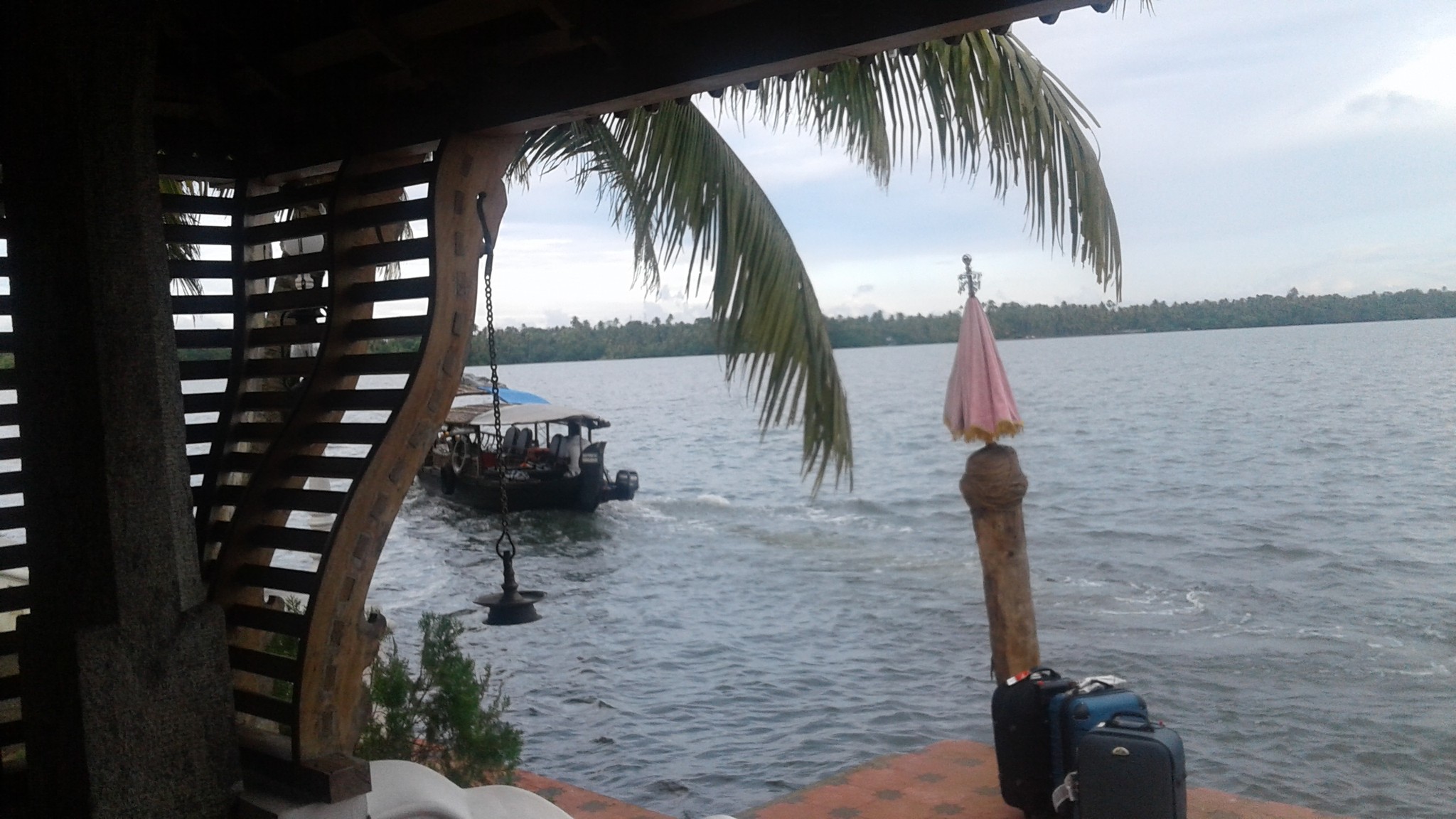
[494,426,522,470]
[497,427,532,473]
[548,434,566,456]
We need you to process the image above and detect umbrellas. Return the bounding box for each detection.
[943,254,1025,445]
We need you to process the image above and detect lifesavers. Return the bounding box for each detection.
[441,468,454,495]
[451,435,472,476]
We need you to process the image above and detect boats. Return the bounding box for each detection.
[411,372,641,516]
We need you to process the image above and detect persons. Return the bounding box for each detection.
[553,422,591,477]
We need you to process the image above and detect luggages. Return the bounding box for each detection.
[1074,711,1187,819]
[1047,679,1147,819]
[990,668,1077,813]
